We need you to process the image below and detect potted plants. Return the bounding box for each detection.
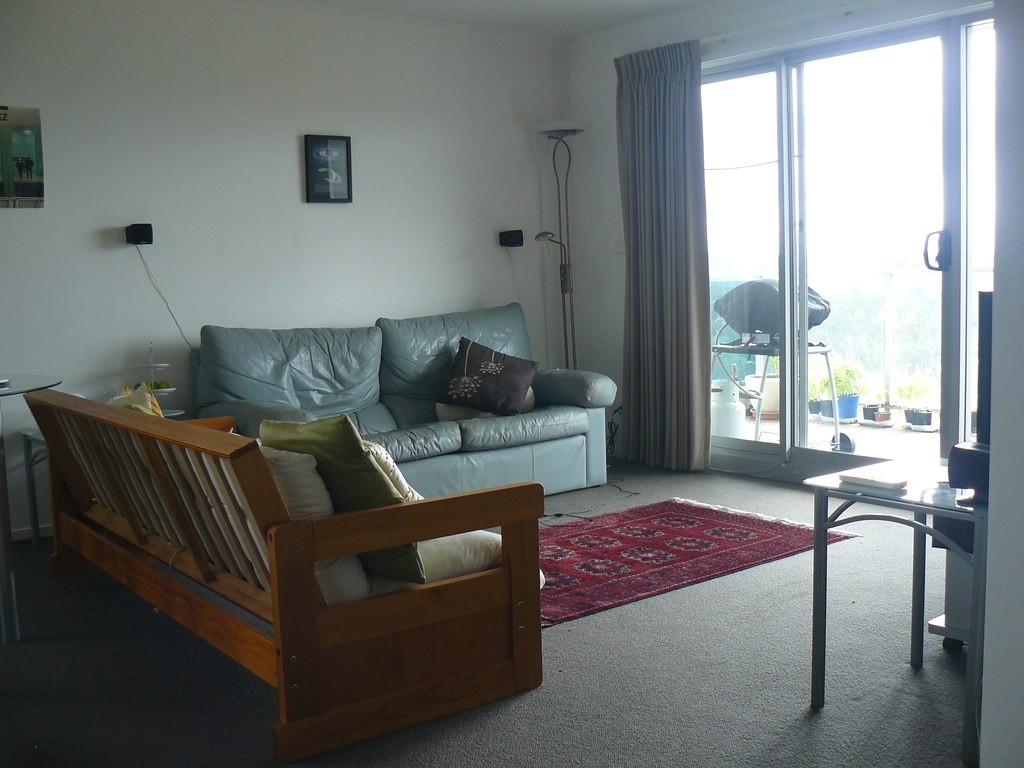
[744,357,780,420]
[820,379,833,417]
[809,381,821,415]
[826,370,862,420]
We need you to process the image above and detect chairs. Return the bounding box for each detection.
[707,279,841,453]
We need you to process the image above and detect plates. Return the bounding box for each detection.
[135,363,170,370]
[152,387,177,396]
[161,409,186,417]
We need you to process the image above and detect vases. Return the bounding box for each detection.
[904,408,932,425]
[863,407,879,420]
[874,412,891,422]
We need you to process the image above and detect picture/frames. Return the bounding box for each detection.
[304,134,352,204]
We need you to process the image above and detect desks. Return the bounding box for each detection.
[801,460,986,710]
[0,372,63,638]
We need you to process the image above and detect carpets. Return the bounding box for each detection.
[537,496,857,628]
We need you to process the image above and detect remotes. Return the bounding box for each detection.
[838,473,908,490]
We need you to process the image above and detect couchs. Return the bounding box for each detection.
[190,301,618,503]
[24,388,545,766]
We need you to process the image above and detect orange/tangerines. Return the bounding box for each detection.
[135,381,169,389]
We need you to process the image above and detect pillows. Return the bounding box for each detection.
[104,382,163,417]
[224,414,428,600]
[436,387,537,423]
[444,337,541,417]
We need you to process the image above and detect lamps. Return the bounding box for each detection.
[534,129,586,372]
